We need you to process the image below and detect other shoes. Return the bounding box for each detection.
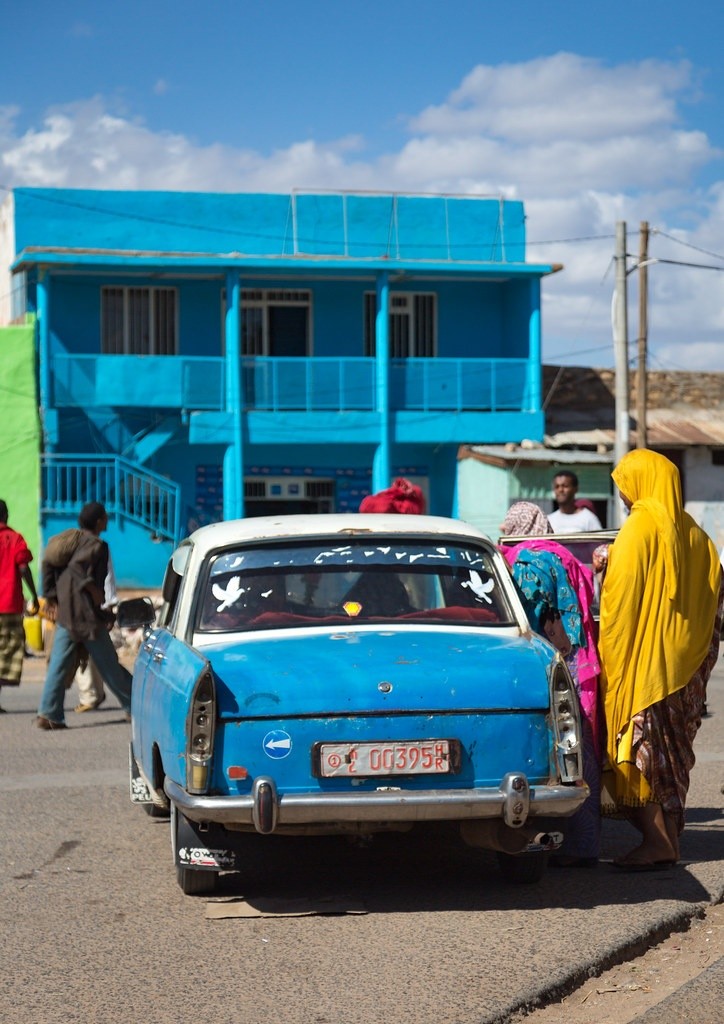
[124,714,132,722]
[31,716,67,732]
[74,691,106,712]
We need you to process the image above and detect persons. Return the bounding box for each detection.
[38,501,133,730]
[495,448,724,872]
[0,500,39,713]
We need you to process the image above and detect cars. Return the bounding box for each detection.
[118,513,619,893]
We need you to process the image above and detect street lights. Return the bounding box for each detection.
[610,256,659,527]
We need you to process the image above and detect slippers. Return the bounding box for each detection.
[548,855,598,869]
[607,852,672,871]
